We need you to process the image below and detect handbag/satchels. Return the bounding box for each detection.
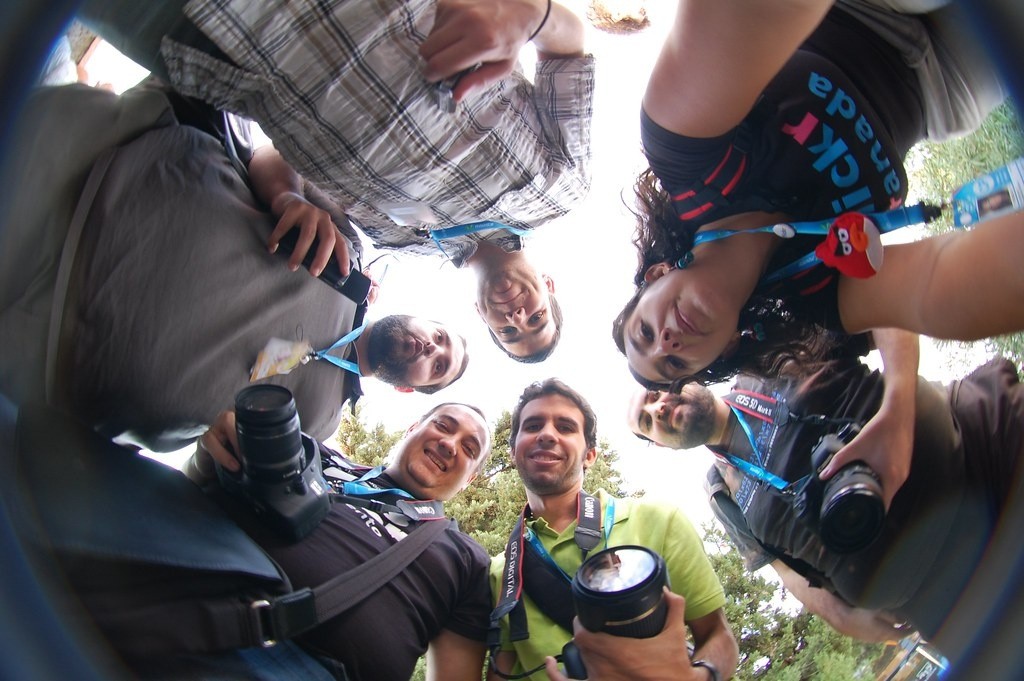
[16,403,293,681]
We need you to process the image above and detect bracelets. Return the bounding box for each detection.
[528,0,552,41]
[692,659,721,681]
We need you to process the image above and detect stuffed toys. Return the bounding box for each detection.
[814,210,883,279]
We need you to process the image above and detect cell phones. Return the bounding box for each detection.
[277,226,372,304]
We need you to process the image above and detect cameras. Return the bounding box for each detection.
[215,382,332,544]
[563,545,671,681]
[793,423,888,554]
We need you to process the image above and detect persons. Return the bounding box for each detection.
[613,0,1024,391]
[627,329,1024,681]
[583,551,621,590]
[977,191,1012,217]
[0,70,468,452]
[71,0,596,363]
[484,379,741,681]
[67,401,491,681]
